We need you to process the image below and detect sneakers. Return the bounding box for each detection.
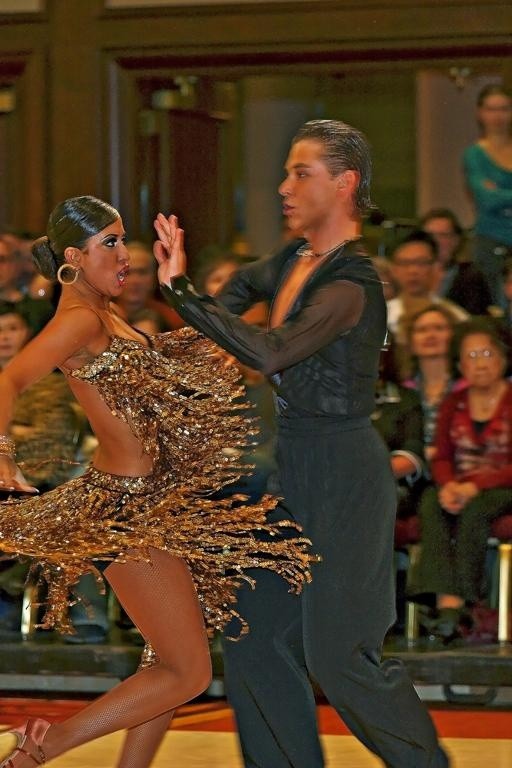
[59,623,107,645]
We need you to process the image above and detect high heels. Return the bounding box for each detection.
[1,717,51,768]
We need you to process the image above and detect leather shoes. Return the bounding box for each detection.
[121,627,146,645]
[426,599,469,638]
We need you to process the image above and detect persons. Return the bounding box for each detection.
[0,192,317,768]
[1,86,510,647]
[150,117,455,767]
